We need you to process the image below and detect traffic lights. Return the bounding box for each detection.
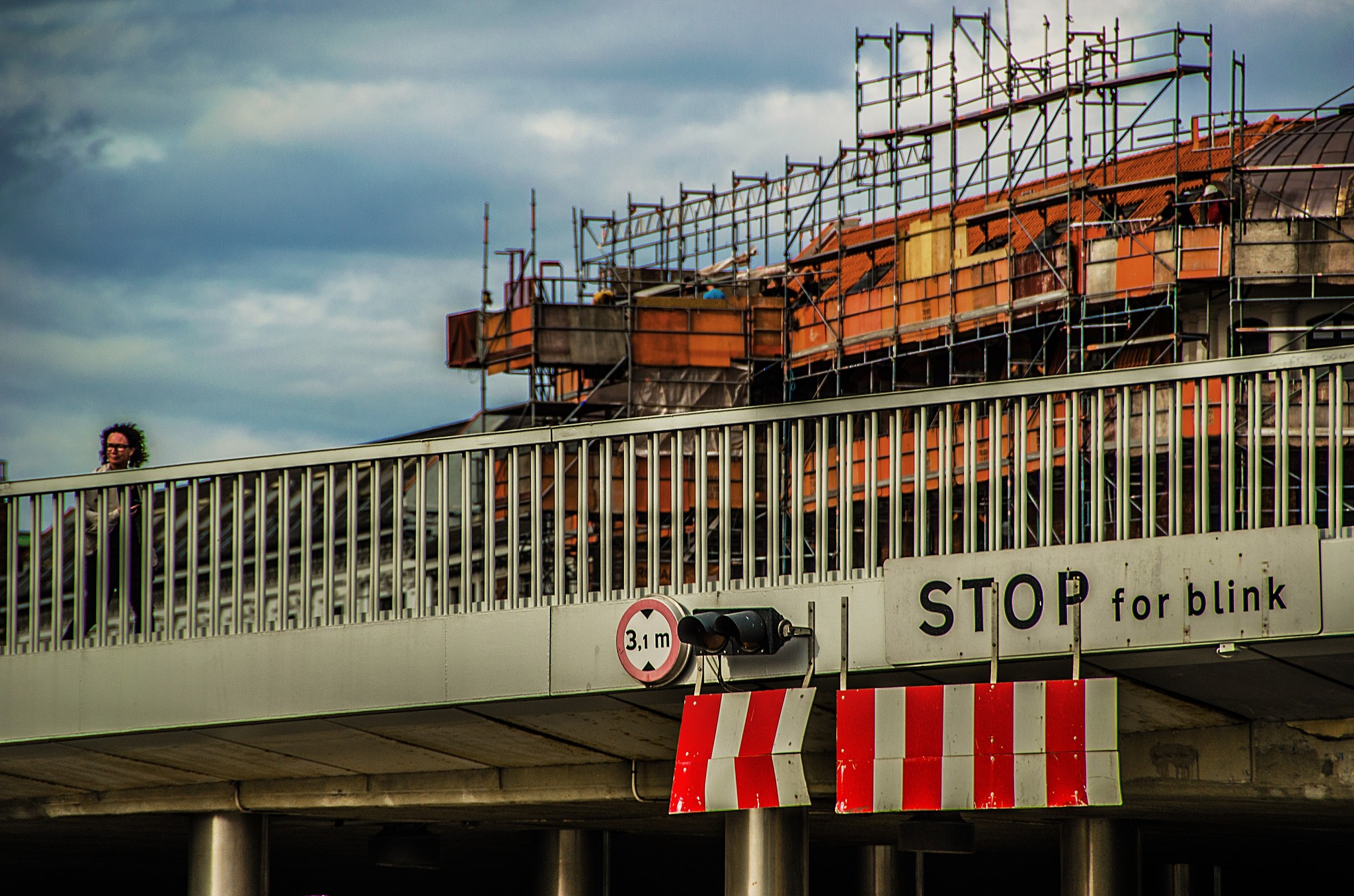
[677,606,793,656]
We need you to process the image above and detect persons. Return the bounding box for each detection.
[1144,190,1196,230]
[1203,184,1229,225]
[703,285,727,299]
[591,290,616,305]
[62,423,159,641]
[798,271,823,305]
[760,277,799,308]
[1096,201,1126,235]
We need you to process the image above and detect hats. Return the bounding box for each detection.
[1203,185,1218,197]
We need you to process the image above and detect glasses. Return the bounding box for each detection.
[107,444,128,451]
